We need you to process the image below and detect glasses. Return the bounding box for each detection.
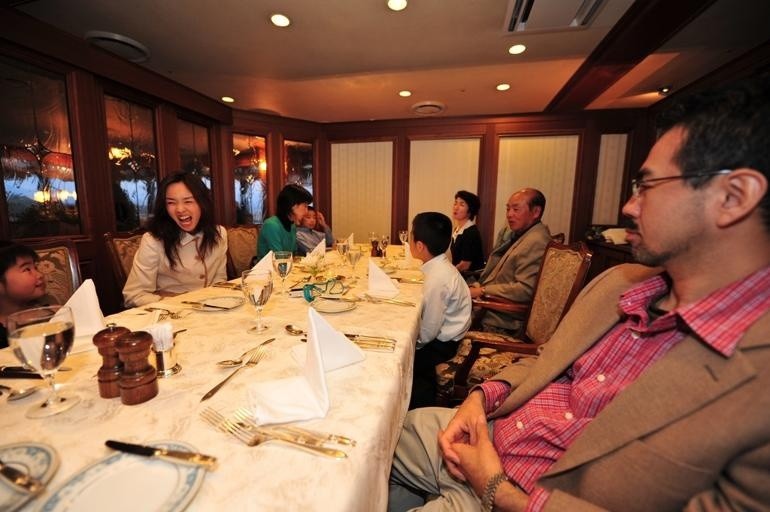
[631,171,736,196]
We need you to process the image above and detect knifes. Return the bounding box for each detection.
[105,439,217,468]
[301,333,396,352]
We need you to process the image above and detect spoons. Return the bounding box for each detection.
[0,383,38,401]
[285,324,308,336]
[215,337,276,368]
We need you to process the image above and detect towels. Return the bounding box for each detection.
[49,280,162,356]
[251,250,276,276]
[245,305,366,426]
[299,237,326,264]
[404,241,411,258]
[365,258,400,299]
[343,232,355,247]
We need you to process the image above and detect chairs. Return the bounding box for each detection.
[221,223,263,279]
[15,237,82,306]
[104,229,144,291]
[472,232,565,315]
[436,239,594,408]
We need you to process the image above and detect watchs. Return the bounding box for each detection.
[482,287,486,296]
[480,471,508,511]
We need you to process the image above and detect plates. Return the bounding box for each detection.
[311,298,357,313]
[42,439,208,512]
[192,296,246,312]
[0,441,60,511]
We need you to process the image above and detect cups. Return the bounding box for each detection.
[346,245,362,275]
[272,251,293,297]
[399,230,409,257]
[337,243,350,266]
[369,232,380,256]
[381,235,389,258]
[8,304,81,420]
[241,268,273,335]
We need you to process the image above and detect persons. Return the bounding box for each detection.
[449,191,485,278]
[256,184,313,261]
[410,213,471,407]
[470,188,554,333]
[297,205,333,254]
[122,170,228,308]
[372,70,770,512]
[0,240,56,349]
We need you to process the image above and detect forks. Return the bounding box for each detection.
[201,345,268,402]
[216,420,345,458]
[199,407,322,448]
[234,407,356,448]
